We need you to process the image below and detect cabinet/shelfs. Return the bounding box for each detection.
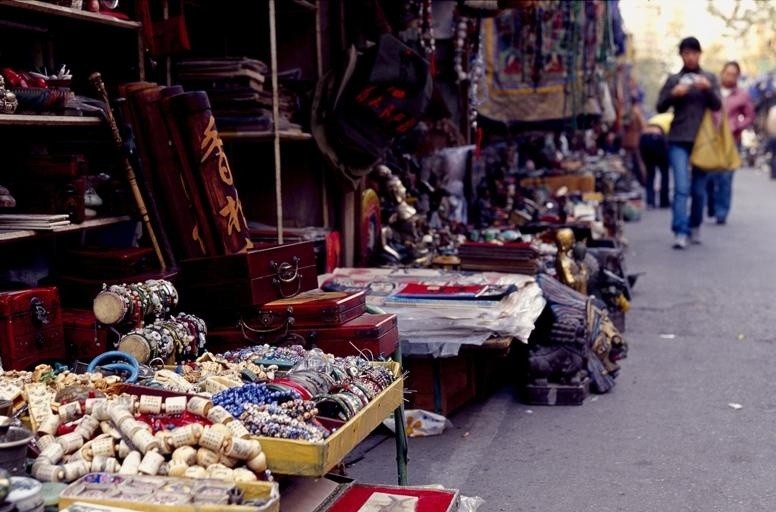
[0,0,145,243]
[162,1,327,248]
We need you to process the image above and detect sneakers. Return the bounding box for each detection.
[671,227,702,249]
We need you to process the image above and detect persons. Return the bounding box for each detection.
[382,176,416,221]
[739,123,759,168]
[749,81,776,180]
[635,109,678,210]
[701,60,756,226]
[551,227,589,297]
[376,209,409,263]
[514,135,583,171]
[621,94,645,187]
[652,34,722,250]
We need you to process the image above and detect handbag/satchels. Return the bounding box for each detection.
[689,102,742,172]
